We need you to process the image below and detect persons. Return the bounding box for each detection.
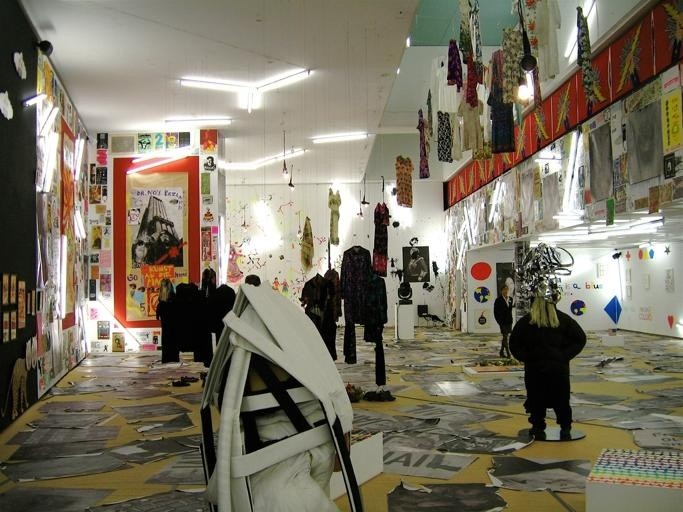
[131,229,181,267]
[406,248,428,281]
[128,209,139,224]
[494,286,514,359]
[508,288,587,439]
[204,156,215,170]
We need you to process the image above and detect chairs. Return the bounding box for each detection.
[418,304,443,327]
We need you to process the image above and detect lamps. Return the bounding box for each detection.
[241,89,402,230]
[164,119,232,127]
[455,129,663,246]
[177,80,240,93]
[217,217,231,287]
[20,92,88,321]
[255,68,311,93]
